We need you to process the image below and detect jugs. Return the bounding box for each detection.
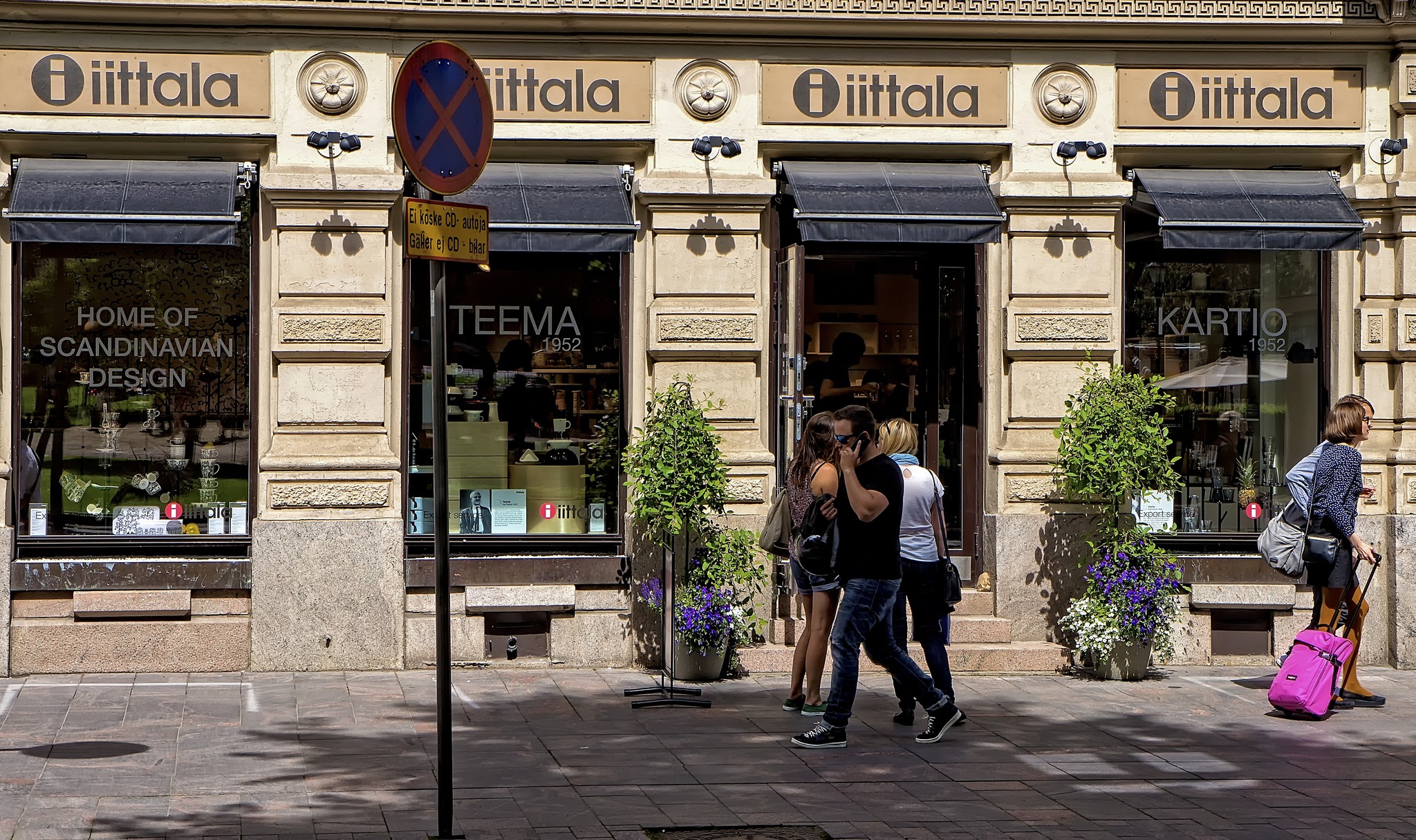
[488,403,499,422]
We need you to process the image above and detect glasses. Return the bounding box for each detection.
[832,432,858,445]
[470,495,482,499]
[1362,416,1369,424]
[883,417,896,436]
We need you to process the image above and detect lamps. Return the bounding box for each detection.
[308,131,361,158]
[1057,141,1107,161]
[1380,138,1407,156]
[691,136,741,161]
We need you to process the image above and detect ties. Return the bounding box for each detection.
[474,507,479,532]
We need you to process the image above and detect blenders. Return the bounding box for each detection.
[1209,467,1224,503]
[1233,398,1248,432]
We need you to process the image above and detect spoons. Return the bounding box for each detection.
[84,480,91,489]
[91,484,118,488]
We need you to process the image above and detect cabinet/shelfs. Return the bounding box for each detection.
[531,368,618,414]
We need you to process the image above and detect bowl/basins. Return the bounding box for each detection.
[166,459,190,470]
[447,387,463,413]
[551,442,570,449]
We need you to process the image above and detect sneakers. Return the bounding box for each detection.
[802,701,828,716]
[782,694,805,711]
[791,721,847,749]
[915,701,962,743]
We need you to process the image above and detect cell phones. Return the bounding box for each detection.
[849,433,871,458]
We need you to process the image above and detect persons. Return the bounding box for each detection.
[19,419,43,518]
[875,417,966,726]
[1277,393,1375,670]
[791,408,963,750]
[1306,403,1386,711]
[460,491,491,534]
[783,410,842,716]
[802,331,921,442]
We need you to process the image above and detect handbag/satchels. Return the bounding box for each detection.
[1257,499,1307,579]
[1301,535,1340,565]
[19,493,30,522]
[758,457,795,559]
[909,613,951,647]
[938,556,963,607]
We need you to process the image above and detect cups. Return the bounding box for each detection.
[460,389,477,399]
[131,471,162,495]
[1201,520,1212,532]
[451,363,463,375]
[59,473,86,503]
[111,413,120,422]
[80,372,90,381]
[867,382,879,403]
[468,414,483,422]
[86,503,105,522]
[160,491,170,503]
[199,449,219,503]
[170,438,183,445]
[533,352,585,410]
[143,408,160,428]
[553,419,571,432]
[1223,489,1235,502]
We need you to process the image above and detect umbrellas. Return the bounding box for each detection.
[1147,352,1288,416]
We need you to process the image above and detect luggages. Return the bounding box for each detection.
[1268,551,1382,719]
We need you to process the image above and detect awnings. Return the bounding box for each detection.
[773,157,1007,245]
[406,157,641,254]
[1127,165,1364,253]
[1,153,247,248]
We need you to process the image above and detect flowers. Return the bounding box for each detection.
[639,547,752,658]
[1057,537,1185,671]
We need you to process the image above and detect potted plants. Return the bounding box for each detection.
[1046,348,1192,682]
[620,374,774,681]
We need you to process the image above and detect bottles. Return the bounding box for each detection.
[1183,495,1200,532]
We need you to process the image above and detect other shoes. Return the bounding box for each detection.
[1276,653,1288,668]
[1339,687,1386,707]
[1329,696,1355,710]
[955,708,966,722]
[893,709,914,726]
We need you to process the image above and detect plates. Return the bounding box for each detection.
[75,380,94,384]
[198,459,218,460]
[198,489,217,490]
[546,440,574,443]
[191,502,226,507]
[464,410,483,413]
[199,478,218,479]
[170,445,186,459]
